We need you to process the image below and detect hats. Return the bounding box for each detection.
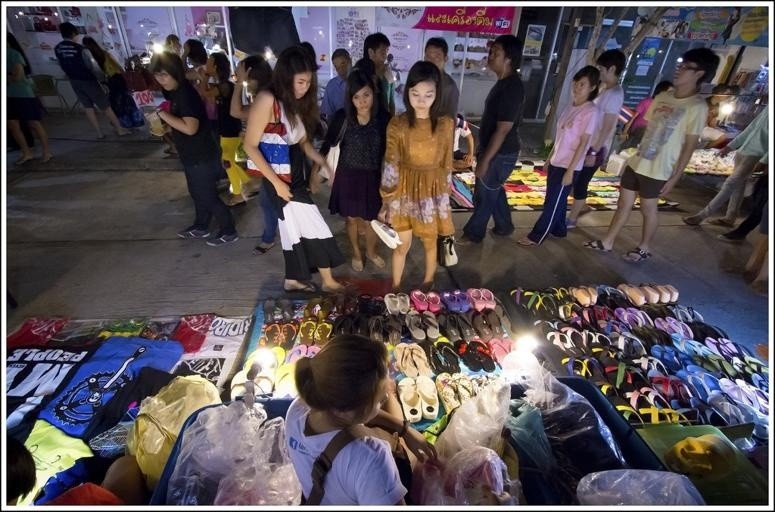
[665,434,738,482]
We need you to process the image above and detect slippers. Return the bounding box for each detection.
[712,218,729,225]
[683,216,698,224]
[371,219,399,248]
[624,247,651,262]
[253,242,275,255]
[584,239,611,252]
[510,284,768,439]
[164,149,178,154]
[14,154,35,165]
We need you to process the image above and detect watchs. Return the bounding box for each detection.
[156,108,162,115]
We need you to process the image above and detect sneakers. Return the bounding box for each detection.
[566,206,590,229]
[207,233,239,246]
[177,229,210,238]
[719,231,744,241]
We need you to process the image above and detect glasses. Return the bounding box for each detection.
[675,65,697,73]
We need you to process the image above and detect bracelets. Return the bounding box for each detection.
[587,147,599,156]
[400,420,410,438]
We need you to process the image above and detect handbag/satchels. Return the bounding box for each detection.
[318,141,341,179]
[123,55,151,91]
[438,237,458,267]
[246,122,292,183]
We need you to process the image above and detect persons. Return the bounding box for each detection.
[345,32,390,111]
[8,434,146,504]
[377,62,455,293]
[425,37,459,118]
[682,106,767,241]
[705,85,740,125]
[163,35,254,208]
[615,81,674,155]
[242,46,352,293]
[144,52,239,247]
[453,112,475,171]
[284,334,436,504]
[383,53,400,112]
[229,55,278,255]
[54,22,133,139]
[83,36,141,127]
[583,48,720,262]
[516,65,601,248]
[321,49,352,126]
[309,73,385,272]
[7,45,54,164]
[565,49,626,228]
[454,34,525,247]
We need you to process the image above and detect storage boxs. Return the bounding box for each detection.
[509,376,673,505]
